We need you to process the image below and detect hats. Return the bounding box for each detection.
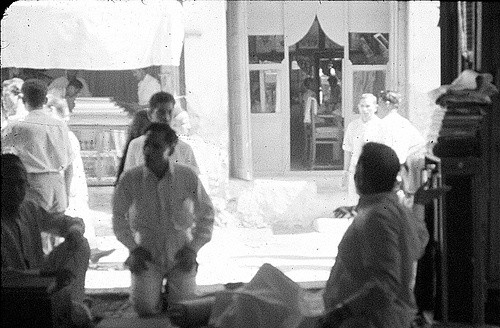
[21,80,48,96]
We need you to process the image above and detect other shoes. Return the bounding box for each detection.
[91,248,115,263]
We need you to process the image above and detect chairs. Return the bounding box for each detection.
[309,99,344,170]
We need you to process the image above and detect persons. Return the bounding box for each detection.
[1,64,444,328]
[111,123,213,315]
